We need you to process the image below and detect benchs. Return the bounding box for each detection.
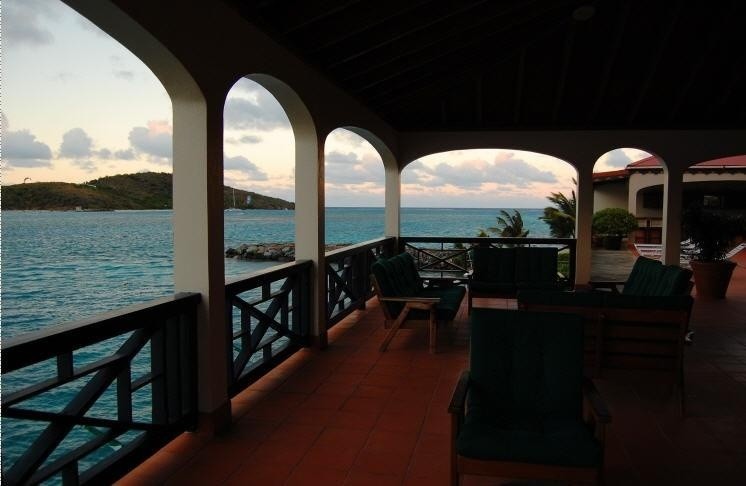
[468,246,563,315]
[369,252,466,353]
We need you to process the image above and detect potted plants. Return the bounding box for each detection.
[679,206,746,298]
[592,207,638,250]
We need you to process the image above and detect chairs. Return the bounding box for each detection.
[588,256,694,387]
[447,307,613,485]
